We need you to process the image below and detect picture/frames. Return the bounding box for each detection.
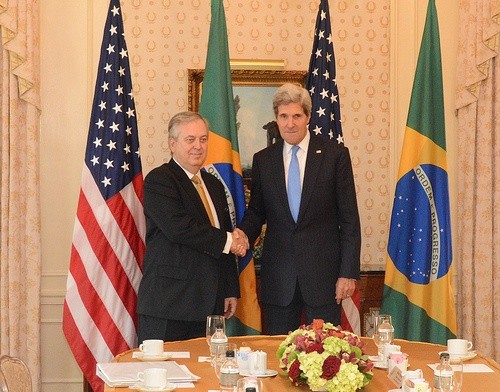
[187,69,307,205]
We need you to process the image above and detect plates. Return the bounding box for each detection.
[368,356,410,369]
[438,351,477,362]
[238,369,279,377]
[133,383,176,392]
[134,354,171,360]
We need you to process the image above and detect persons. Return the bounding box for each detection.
[136,111,248,347]
[230,84,362,335]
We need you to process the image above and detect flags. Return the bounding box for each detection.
[308,0,361,338]
[62,1,146,391]
[200,0,261,338]
[384,0,459,345]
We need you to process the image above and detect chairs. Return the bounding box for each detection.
[0,354,33,392]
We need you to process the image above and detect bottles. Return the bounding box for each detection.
[434,353,454,392]
[220,350,240,392]
[210,324,228,367]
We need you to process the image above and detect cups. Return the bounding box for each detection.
[139,339,163,356]
[447,339,473,354]
[387,358,408,375]
[136,368,167,387]
[236,376,264,392]
[373,316,394,347]
[235,351,267,375]
[215,343,237,380]
[439,357,463,392]
[400,371,431,392]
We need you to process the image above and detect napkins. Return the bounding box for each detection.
[129,383,194,389]
[198,356,211,362]
[208,390,221,392]
[427,363,494,372]
[132,352,190,359]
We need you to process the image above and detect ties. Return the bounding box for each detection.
[191,175,215,227]
[288,146,301,224]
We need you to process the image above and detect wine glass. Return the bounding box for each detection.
[205,315,225,360]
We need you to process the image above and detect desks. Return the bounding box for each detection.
[104,335,500,392]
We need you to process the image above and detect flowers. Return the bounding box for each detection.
[276,319,374,392]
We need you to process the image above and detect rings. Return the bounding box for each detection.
[347,295,349,296]
